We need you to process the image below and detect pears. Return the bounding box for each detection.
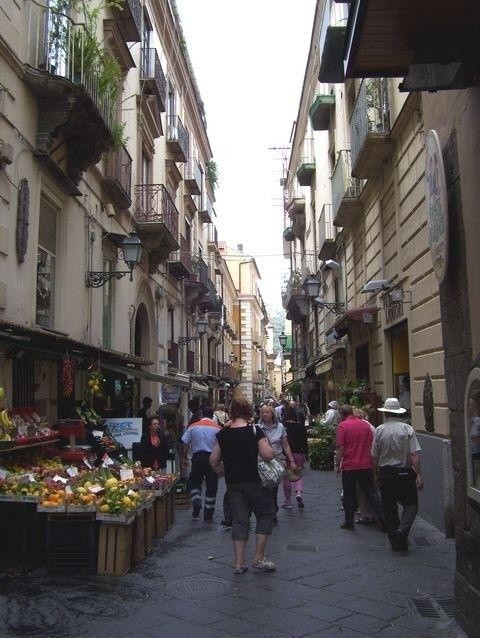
[100,504,109,512]
[123,497,131,504]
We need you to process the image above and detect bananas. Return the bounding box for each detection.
[0,409,13,427]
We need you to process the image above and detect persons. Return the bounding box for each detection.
[369,398,422,551]
[335,403,384,529]
[467,396,479,483]
[138,397,170,475]
[209,398,279,574]
[251,397,307,525]
[322,401,341,426]
[180,399,232,527]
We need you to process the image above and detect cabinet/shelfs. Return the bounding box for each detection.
[342,388,384,428]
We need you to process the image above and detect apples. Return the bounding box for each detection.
[43,494,62,506]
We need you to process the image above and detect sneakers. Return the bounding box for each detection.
[233,561,249,573]
[281,502,293,508]
[192,511,199,520]
[391,529,408,552]
[222,518,232,525]
[296,494,304,508]
[341,522,354,528]
[253,557,278,569]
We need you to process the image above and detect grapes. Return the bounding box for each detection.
[43,468,79,489]
[136,479,167,489]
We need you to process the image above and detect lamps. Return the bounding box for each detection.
[302,277,344,316]
[279,331,301,354]
[85,231,145,288]
[177,315,208,350]
[217,351,237,369]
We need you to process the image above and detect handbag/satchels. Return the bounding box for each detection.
[287,466,303,482]
[257,458,286,487]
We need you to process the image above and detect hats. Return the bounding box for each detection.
[329,400,337,408]
[377,397,407,414]
